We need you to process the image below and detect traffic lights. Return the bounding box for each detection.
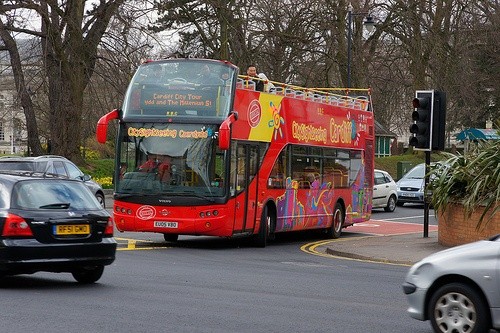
[410,91,432,149]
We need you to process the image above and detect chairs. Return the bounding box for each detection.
[169,77,371,112]
[289,166,359,190]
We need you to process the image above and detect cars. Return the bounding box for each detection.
[373,169,398,211]
[0,170,117,282]
[397,163,453,208]
[403,233,500,333]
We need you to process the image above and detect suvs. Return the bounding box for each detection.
[2,155,106,210]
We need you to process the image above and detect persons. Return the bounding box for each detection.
[121,152,321,189]
[145,62,232,82]
[259,72,276,93]
[243,64,264,91]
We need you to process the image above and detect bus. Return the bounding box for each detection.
[96,58,375,248]
